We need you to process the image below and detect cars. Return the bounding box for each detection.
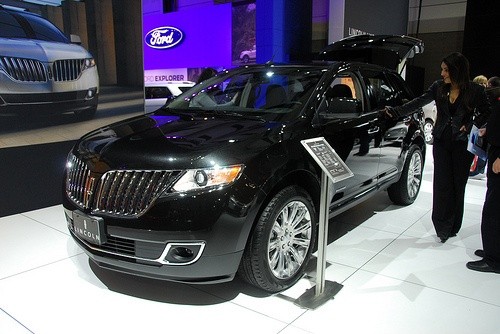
[144,80,196,115]
[59,34,428,294]
[0,4,100,130]
[240,45,256,64]
[421,100,438,145]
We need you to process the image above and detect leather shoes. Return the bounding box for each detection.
[475,250,484,257]
[467,260,500,274]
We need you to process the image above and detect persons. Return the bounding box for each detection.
[384,52,489,243]
[473,75,487,87]
[190,69,239,107]
[466,76,500,274]
